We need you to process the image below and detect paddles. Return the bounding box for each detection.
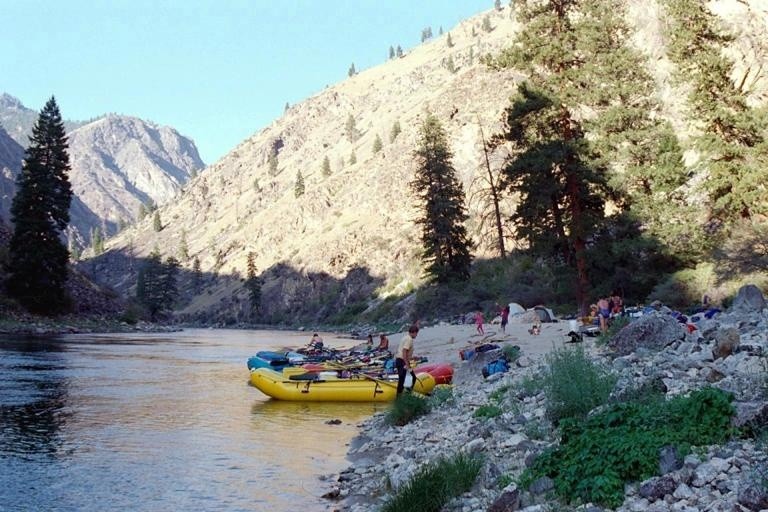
[357,372,427,399]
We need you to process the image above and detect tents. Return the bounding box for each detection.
[527,304,559,322]
[508,302,525,323]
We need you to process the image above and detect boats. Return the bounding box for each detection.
[246,350,455,402]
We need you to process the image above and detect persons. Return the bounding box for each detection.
[396,326,419,399]
[496,303,510,334]
[471,313,484,335]
[375,332,388,351]
[368,335,373,346]
[590,294,623,337]
[655,300,671,314]
[308,333,323,353]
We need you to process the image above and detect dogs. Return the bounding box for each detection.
[564,330,583,343]
